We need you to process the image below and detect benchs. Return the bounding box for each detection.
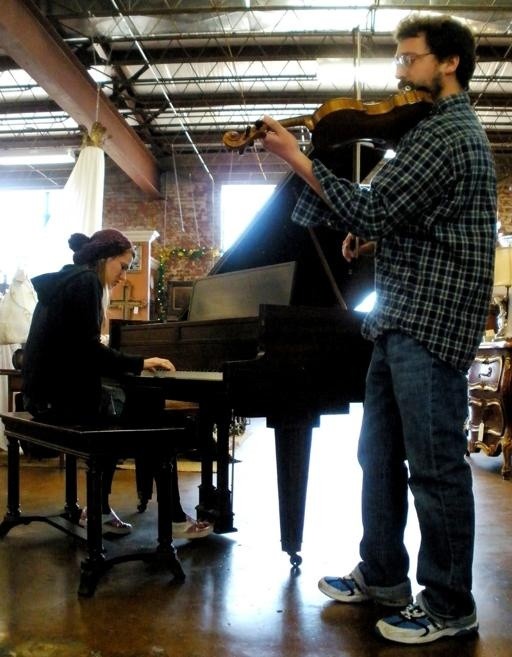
[0,409,187,596]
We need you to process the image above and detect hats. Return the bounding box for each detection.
[68,228,132,264]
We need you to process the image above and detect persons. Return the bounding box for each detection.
[20,229,213,540]
[259,15,498,645]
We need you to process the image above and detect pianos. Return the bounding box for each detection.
[109,138,386,567]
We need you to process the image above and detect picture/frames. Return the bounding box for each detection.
[126,245,143,273]
[167,279,194,315]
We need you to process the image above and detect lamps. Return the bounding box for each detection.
[493,243,511,342]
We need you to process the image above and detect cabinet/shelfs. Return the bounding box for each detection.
[467,341,511,480]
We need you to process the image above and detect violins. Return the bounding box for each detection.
[222,90,436,155]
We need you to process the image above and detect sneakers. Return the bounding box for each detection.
[172,513,214,539]
[375,598,481,643]
[318,574,417,609]
[78,504,133,536]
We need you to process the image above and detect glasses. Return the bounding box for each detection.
[393,52,436,67]
